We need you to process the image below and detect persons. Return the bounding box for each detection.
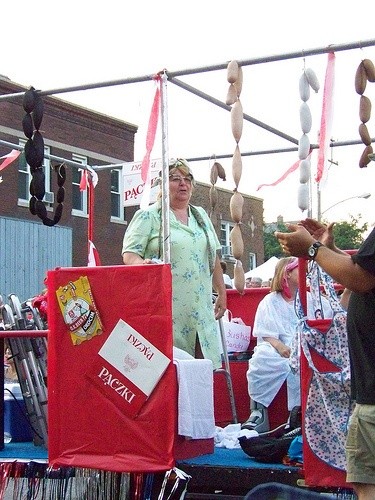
[121,159,227,371]
[274,218,375,500]
[242,256,334,433]
[221,262,272,288]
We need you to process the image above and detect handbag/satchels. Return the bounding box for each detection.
[217,307,251,355]
[287,435,304,463]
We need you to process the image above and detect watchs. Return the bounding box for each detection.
[306,241,325,260]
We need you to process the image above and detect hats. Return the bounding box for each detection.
[159,158,197,187]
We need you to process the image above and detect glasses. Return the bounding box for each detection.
[171,176,192,184]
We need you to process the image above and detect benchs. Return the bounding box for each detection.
[214,283,344,435]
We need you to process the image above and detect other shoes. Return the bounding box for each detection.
[286,405,302,430]
[239,399,270,433]
